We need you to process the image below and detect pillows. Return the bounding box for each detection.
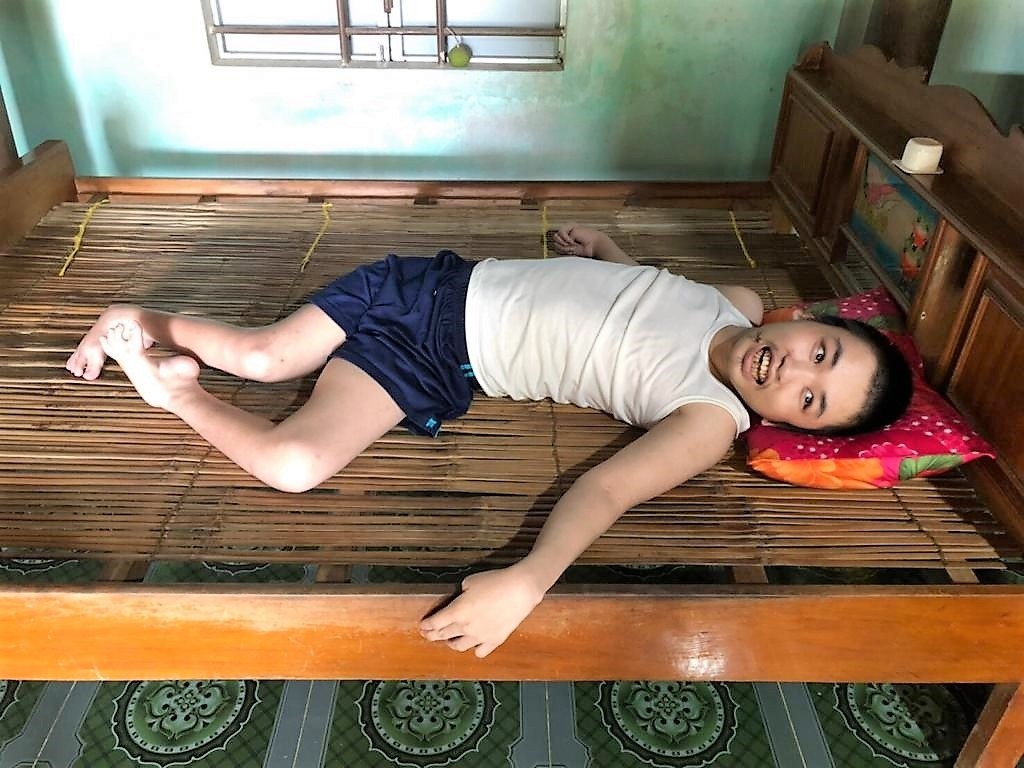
[747,288,996,489]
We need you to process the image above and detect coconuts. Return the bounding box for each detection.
[449,46,471,67]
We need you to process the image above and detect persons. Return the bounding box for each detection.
[64,224,913,657]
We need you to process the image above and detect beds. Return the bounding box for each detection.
[0,38,1024,768]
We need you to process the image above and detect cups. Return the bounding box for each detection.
[902,137,943,171]
